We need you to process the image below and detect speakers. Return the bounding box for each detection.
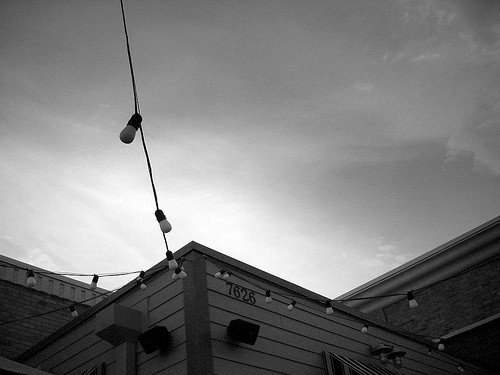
[137,326,173,355]
[227,318,260,345]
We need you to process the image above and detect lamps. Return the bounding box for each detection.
[387,347,407,369]
[370,341,392,365]
